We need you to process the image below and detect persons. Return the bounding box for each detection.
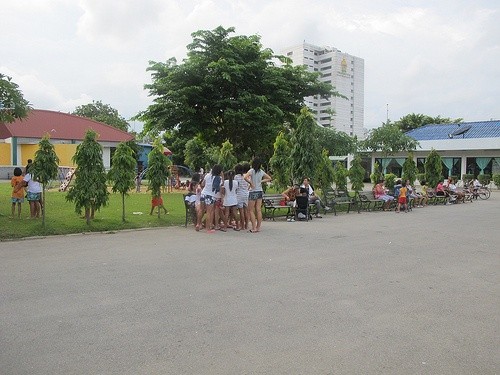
[135,157,331,233]
[375,179,482,213]
[11,160,43,219]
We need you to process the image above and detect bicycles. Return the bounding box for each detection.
[464,181,490,200]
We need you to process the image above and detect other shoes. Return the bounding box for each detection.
[447,202,451,205]
[421,204,423,207]
[316,215,322,218]
[286,216,296,221]
[417,205,419,207]
[324,206,330,211]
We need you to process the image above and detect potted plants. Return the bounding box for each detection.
[478,175,500,189]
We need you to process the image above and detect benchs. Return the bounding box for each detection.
[262,195,316,221]
[183,192,205,227]
[322,187,478,216]
